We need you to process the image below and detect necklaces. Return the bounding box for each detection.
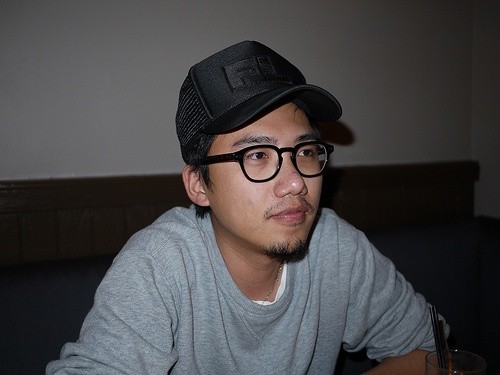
[265,261,283,301]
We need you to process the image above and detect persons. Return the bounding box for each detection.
[45,41,450,375]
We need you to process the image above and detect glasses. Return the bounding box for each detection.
[198,140,334,183]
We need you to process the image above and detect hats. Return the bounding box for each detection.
[175,41,342,159]
